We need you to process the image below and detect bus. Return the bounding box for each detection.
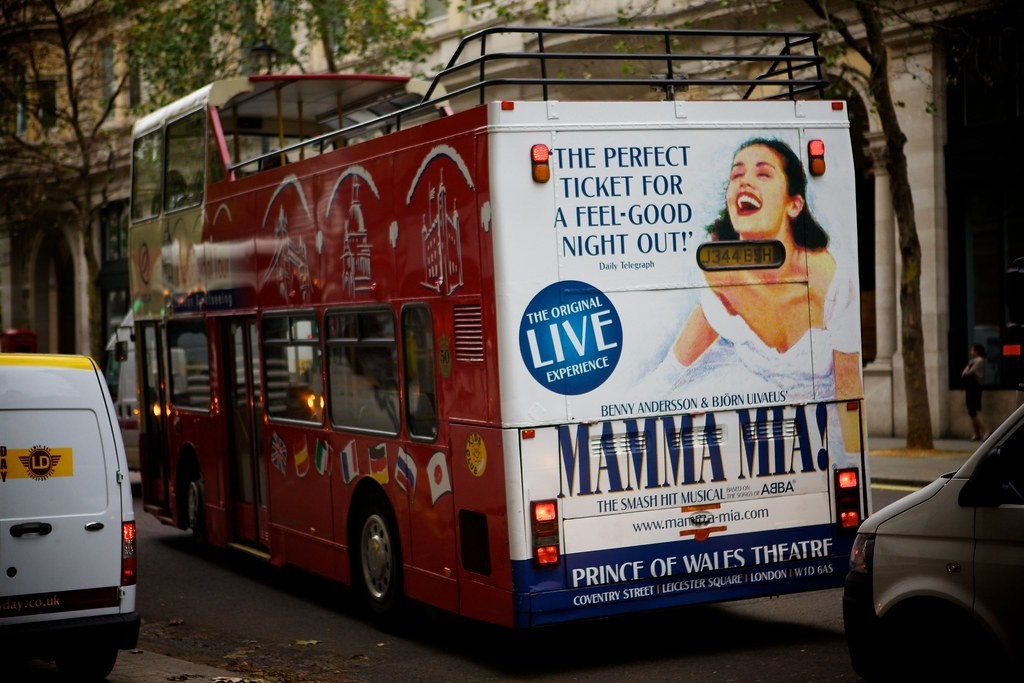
[114,28,872,628]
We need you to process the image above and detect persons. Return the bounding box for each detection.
[626,137,867,457]
[962,343,990,441]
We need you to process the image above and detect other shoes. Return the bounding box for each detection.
[971,435,978,441]
[982,432,989,441]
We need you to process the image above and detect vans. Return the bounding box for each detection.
[843,404,1024,683]
[0,355,141,683]
